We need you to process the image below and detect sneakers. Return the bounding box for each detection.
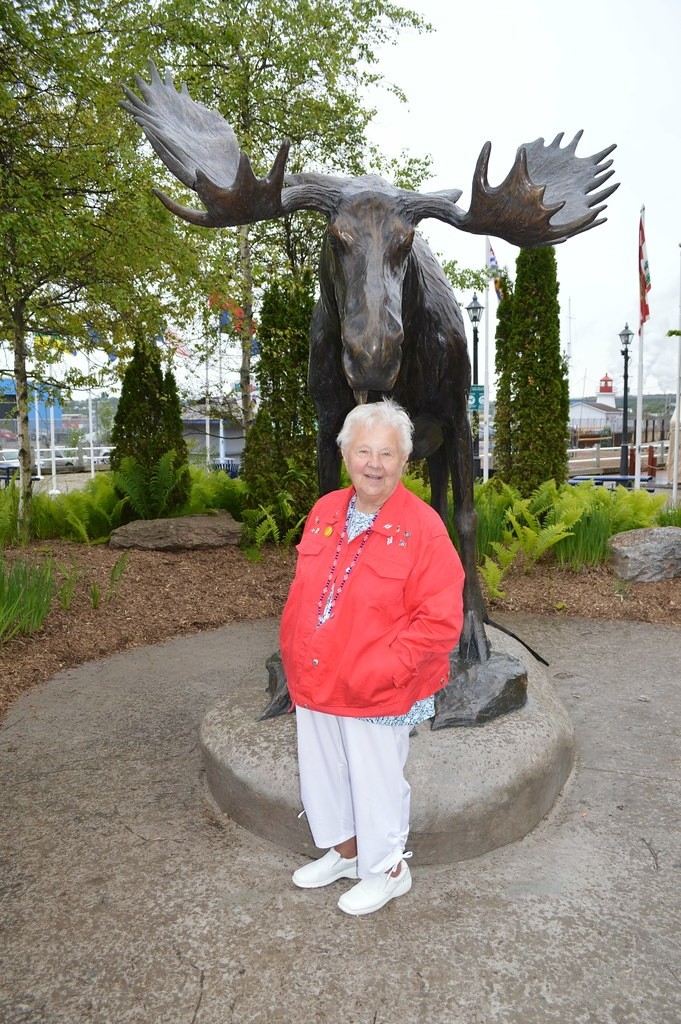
[337,851,413,916]
[291,848,359,888]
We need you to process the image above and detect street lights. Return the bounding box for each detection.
[617,321,635,489]
[464,292,485,487]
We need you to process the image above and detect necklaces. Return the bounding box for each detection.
[316,495,382,629]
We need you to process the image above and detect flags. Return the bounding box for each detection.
[219,298,258,338]
[488,238,504,300]
[87,321,120,362]
[209,282,234,312]
[50,315,77,356]
[247,339,263,356]
[153,322,188,356]
[638,209,652,335]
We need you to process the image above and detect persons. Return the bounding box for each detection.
[279,401,465,918]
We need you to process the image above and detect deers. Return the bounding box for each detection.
[117,56,620,663]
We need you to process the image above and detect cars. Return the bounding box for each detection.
[0,426,118,475]
